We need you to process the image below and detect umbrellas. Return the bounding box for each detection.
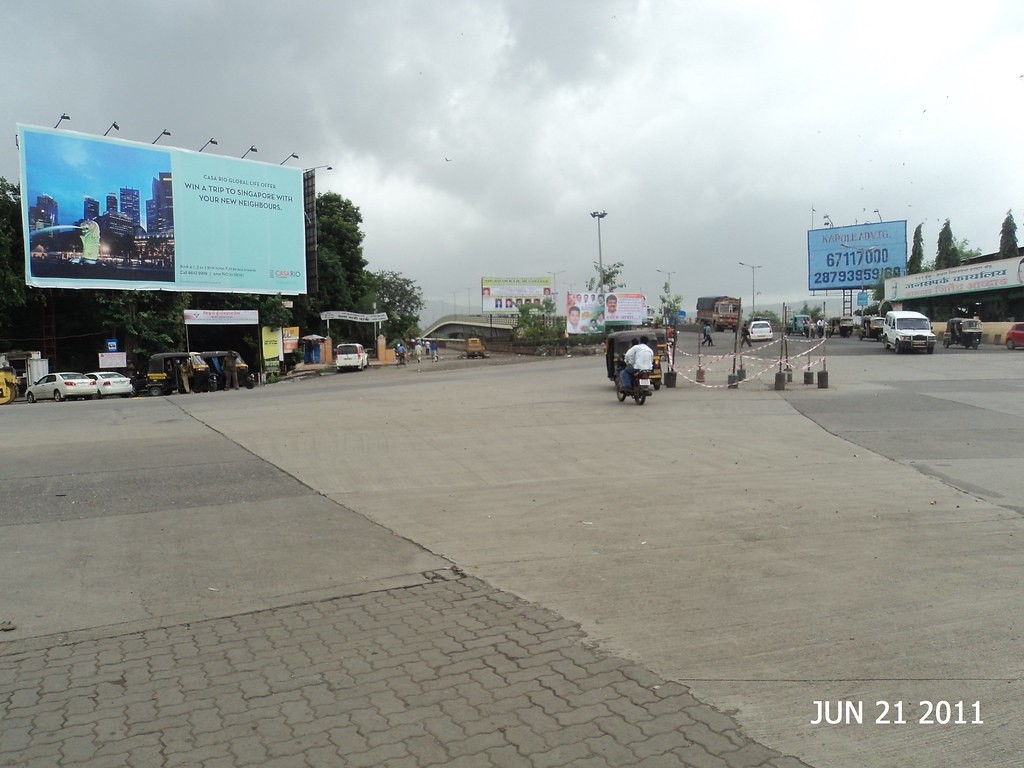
[302,334,327,341]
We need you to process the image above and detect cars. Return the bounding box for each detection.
[68,372,134,401]
[25,372,98,404]
[747,321,773,342]
[1005,323,1024,350]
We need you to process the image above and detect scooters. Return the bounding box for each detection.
[395,348,438,368]
[801,323,831,339]
[943,332,953,349]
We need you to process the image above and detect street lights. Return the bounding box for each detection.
[656,269,676,298]
[547,270,565,300]
[739,262,764,315]
[590,210,609,292]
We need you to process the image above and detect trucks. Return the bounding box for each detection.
[785,314,811,336]
[695,296,743,333]
[465,338,485,359]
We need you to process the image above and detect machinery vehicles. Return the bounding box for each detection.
[0,352,21,405]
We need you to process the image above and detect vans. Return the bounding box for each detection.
[335,343,370,371]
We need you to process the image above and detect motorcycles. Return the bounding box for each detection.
[946,318,982,349]
[839,316,854,337]
[195,350,254,390]
[860,317,886,342]
[604,327,673,391]
[147,350,212,396]
[612,353,655,405]
[652,328,669,363]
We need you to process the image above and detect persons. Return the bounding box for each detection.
[563,329,571,357]
[544,288,549,295]
[425,340,437,362]
[621,336,654,392]
[397,344,406,365]
[659,325,674,347]
[568,294,604,333]
[803,317,829,335]
[167,350,240,390]
[701,323,715,346]
[304,340,321,364]
[483,288,489,295]
[1018,257,1024,284]
[606,294,617,313]
[890,280,897,299]
[740,324,752,347]
[415,343,422,363]
[496,299,539,308]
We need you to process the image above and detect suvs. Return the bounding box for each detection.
[882,311,937,355]
